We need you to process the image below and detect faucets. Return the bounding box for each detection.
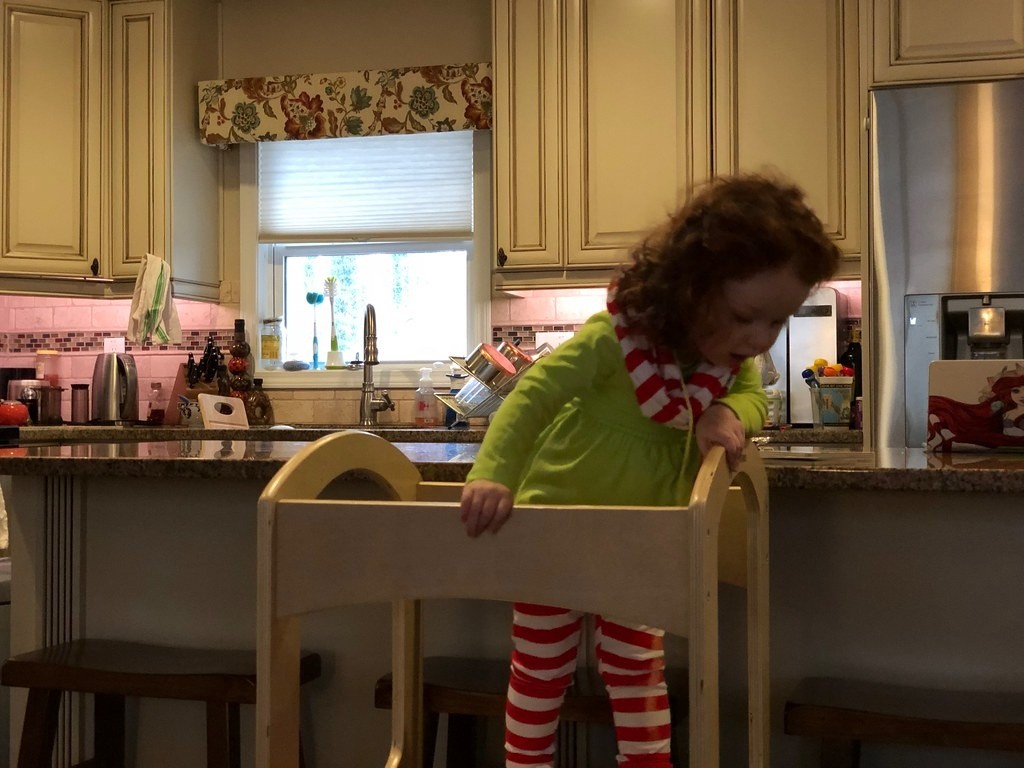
[359,304,397,422]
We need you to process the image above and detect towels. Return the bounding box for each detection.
[126,254,183,348]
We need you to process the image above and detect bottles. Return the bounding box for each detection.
[34,350,60,387]
[258,319,283,371]
[148,382,166,423]
[415,368,437,427]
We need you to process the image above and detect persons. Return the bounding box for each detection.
[462,174,839,768]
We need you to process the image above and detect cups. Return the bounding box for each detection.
[809,387,820,430]
[71,383,90,425]
[819,376,853,430]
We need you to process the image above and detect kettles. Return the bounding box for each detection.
[92,353,137,428]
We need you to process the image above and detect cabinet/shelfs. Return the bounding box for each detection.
[492,0,1024,291]
[0,0,220,301]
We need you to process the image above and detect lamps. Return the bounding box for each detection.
[103,336,126,353]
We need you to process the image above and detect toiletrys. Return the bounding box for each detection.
[413,366,440,428]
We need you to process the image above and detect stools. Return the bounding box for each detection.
[0,638,320,768]
[784,678,1024,768]
[374,657,689,768]
